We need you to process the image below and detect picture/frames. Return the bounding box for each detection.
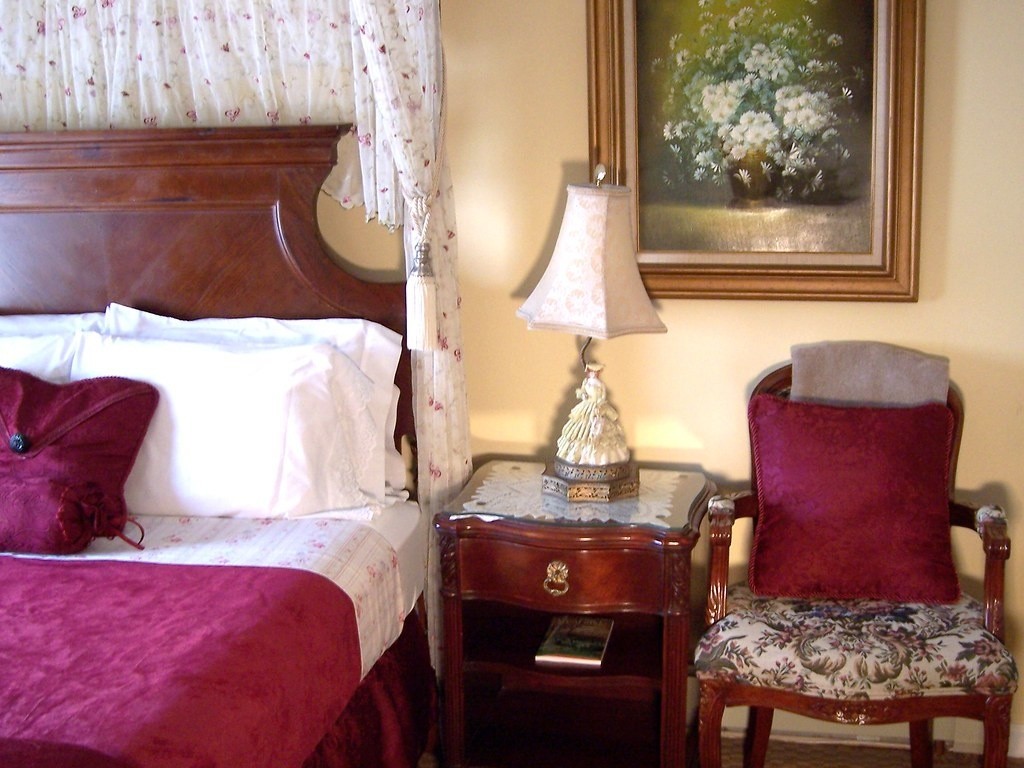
[585,0,925,304]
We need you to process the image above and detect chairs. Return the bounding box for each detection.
[692,343,1018,768]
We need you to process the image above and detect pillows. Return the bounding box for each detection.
[0,302,411,525]
[0,366,159,555]
[747,394,961,605]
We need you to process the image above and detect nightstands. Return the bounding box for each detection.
[432,458,718,768]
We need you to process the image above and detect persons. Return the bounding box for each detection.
[535,617,614,666]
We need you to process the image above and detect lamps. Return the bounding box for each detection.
[518,164,669,505]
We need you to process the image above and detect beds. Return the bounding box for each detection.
[0,124,445,768]
